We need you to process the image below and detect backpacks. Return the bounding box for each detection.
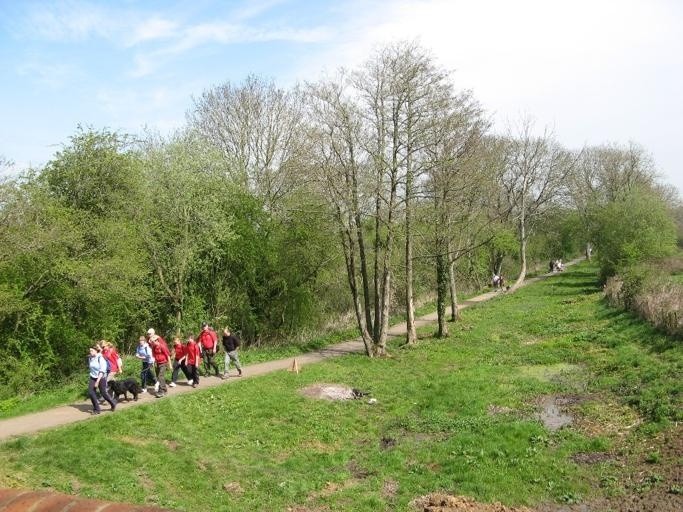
[97,356,110,375]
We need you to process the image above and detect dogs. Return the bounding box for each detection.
[107,377,143,401]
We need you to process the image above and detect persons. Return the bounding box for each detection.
[88,322,220,414]
[548,257,565,273]
[491,274,507,293]
[222,329,243,379]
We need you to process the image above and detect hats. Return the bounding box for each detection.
[147,336,157,343]
[139,336,146,341]
[147,328,155,334]
[202,321,206,326]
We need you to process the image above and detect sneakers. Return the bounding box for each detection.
[142,368,245,398]
[91,394,118,414]
